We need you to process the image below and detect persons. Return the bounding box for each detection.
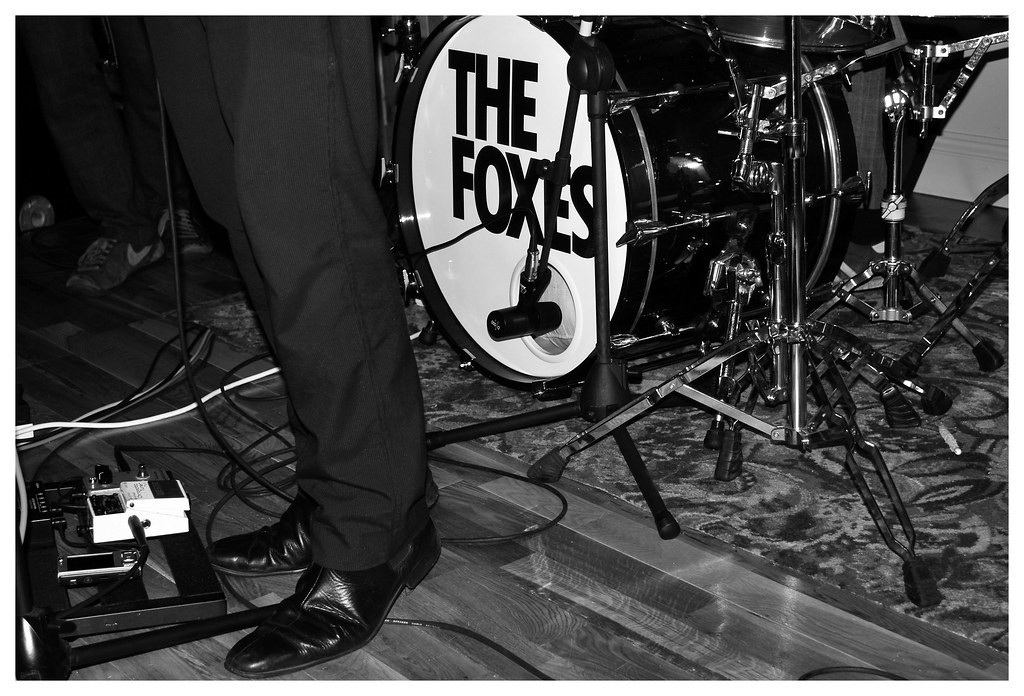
[21,15,212,298]
[146,16,442,677]
[841,59,887,210]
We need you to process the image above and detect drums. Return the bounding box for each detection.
[660,15,888,55]
[378,15,874,403]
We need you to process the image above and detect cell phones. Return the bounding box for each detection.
[56,548,143,585]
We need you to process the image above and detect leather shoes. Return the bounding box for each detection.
[223,517,442,677]
[206,470,440,577]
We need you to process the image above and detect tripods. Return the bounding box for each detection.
[423,16,1002,606]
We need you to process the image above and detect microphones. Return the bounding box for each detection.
[487,268,563,342]
